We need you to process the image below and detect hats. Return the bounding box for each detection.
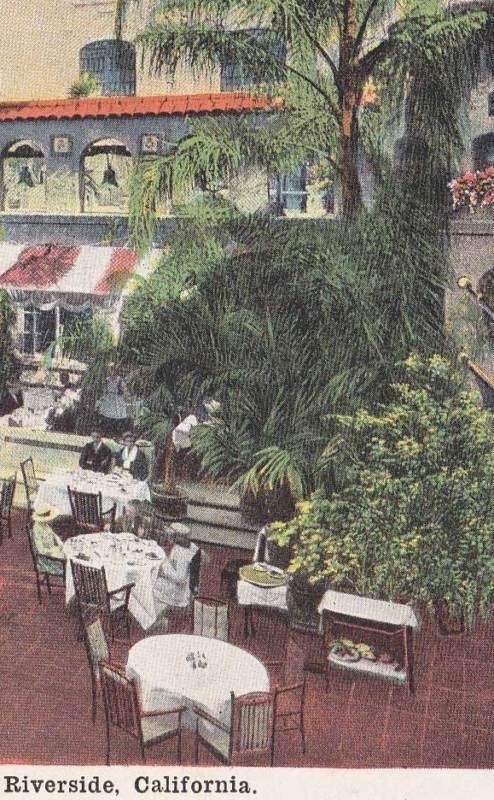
[32,501,61,523]
[168,521,192,536]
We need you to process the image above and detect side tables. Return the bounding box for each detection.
[31,471,157,535]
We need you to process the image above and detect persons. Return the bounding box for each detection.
[77,427,113,475]
[51,370,80,401]
[93,368,132,422]
[113,432,150,482]
[148,520,210,633]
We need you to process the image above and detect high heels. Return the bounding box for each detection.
[152,617,169,632]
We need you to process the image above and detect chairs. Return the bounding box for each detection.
[222,522,422,692]
[63,483,114,534]
[94,651,184,764]
[63,558,133,643]
[72,617,113,726]
[258,640,316,758]
[188,594,227,648]
[15,451,37,503]
[188,685,274,768]
[26,521,67,598]
[167,549,207,626]
[0,470,20,546]
